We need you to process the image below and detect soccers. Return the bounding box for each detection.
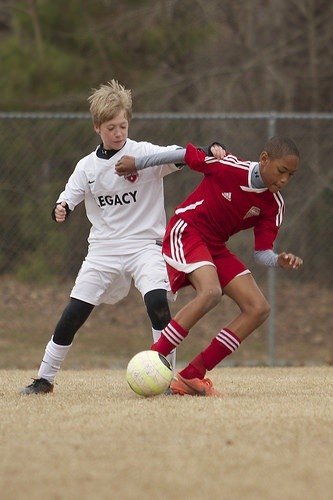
[126,350,173,396]
